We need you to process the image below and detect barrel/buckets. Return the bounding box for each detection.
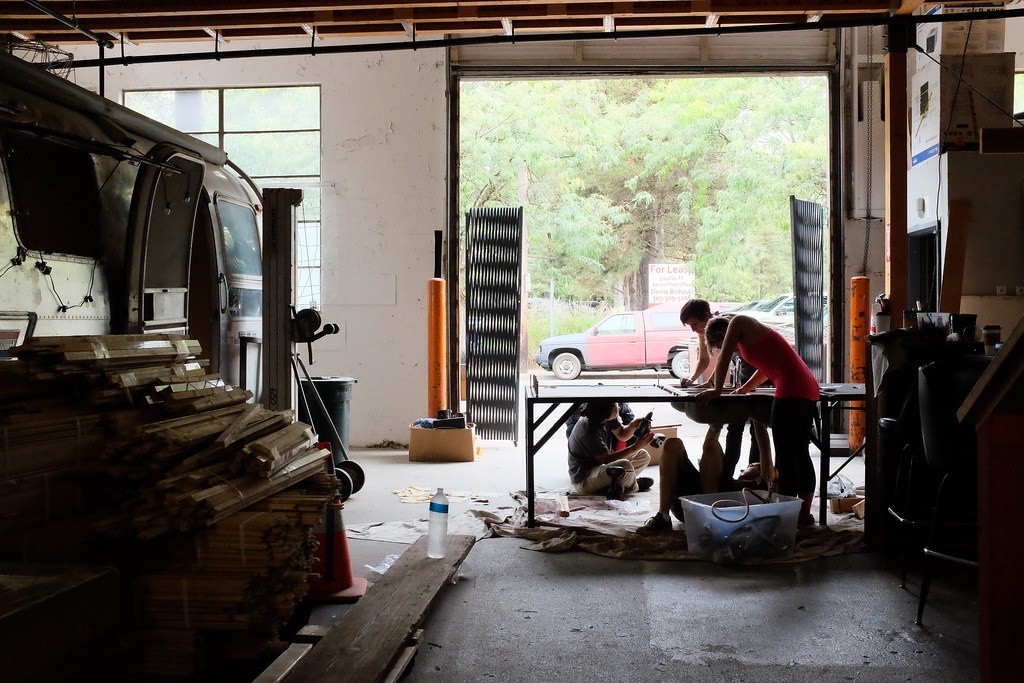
[298,375,358,471]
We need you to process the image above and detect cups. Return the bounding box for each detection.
[983,325,1002,356]
[874,312,891,335]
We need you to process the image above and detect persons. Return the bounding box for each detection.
[566,402,655,495]
[679,298,821,528]
[636,415,779,537]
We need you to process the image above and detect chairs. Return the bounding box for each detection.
[916,463,977,625]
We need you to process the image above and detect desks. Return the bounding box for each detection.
[526,383,866,531]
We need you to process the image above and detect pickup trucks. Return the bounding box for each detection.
[533,309,704,381]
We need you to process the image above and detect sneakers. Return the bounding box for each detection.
[636,512,673,537]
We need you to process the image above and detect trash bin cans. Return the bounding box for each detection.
[301,376,358,469]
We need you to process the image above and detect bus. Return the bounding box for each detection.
[0,46,265,407]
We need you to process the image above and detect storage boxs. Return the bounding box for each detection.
[910,52,1016,166]
[678,490,805,564]
[916,1,1005,73]
[851,499,864,519]
[830,498,864,513]
[408,422,477,461]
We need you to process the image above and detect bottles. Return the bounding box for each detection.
[426,487,449,559]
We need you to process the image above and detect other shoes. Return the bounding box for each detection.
[798,514,814,525]
[637,477,654,491]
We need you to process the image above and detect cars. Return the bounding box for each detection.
[711,293,795,347]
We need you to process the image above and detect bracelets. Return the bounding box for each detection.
[708,378,714,387]
[629,421,639,428]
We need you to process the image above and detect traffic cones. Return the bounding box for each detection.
[316,441,368,600]
